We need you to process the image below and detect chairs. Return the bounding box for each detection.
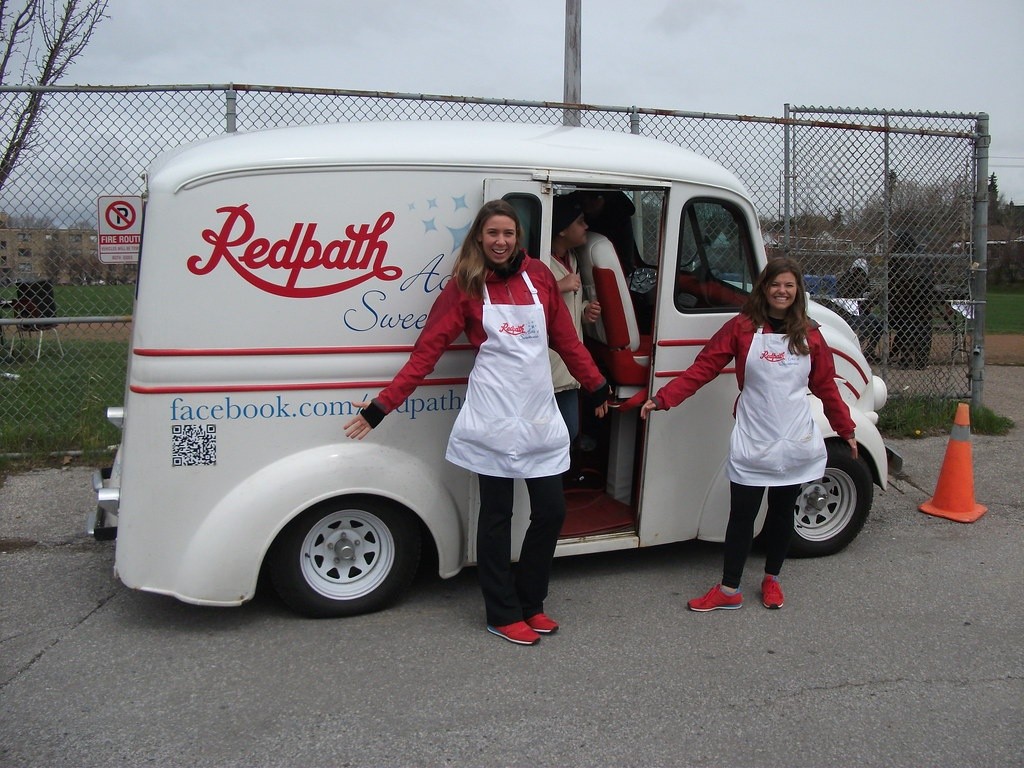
[7,281,64,363]
[577,231,652,385]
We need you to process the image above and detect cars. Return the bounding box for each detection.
[68,276,106,286]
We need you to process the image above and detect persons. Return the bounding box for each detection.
[641,257,858,612]
[837,259,883,363]
[888,232,934,368]
[343,202,613,644]
[551,200,601,440]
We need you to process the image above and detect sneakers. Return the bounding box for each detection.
[485,620,540,645]
[527,610,558,635]
[687,584,743,612]
[761,574,784,609]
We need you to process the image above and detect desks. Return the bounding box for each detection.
[811,297,975,391]
[0,300,13,345]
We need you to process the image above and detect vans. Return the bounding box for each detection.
[93,120,906,617]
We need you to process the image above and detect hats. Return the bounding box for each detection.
[850,258,868,275]
[552,191,581,230]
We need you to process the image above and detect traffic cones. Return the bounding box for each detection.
[917,396,988,520]
